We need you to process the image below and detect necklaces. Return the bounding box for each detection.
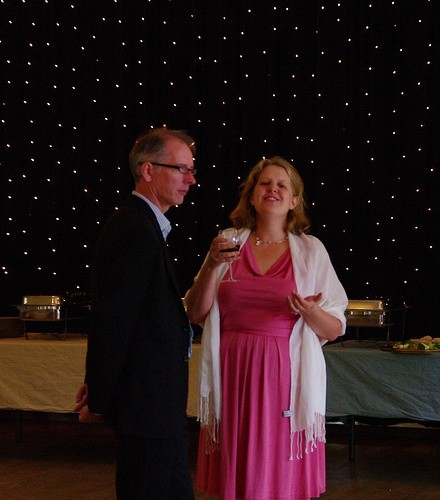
[252,230,288,247]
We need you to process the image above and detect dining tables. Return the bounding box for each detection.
[1,332,202,442]
[322,340,440,463]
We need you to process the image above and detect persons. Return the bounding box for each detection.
[184,156,349,500]
[74,129,196,500]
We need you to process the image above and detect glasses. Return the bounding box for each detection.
[138,162,196,174]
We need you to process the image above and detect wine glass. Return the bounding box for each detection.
[218,230,241,283]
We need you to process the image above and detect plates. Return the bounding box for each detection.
[387,346,440,354]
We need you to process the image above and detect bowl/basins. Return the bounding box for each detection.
[0,318,24,337]
